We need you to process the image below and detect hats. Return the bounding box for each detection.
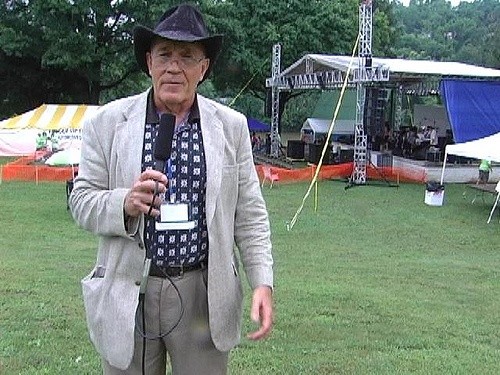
[133,4,224,85]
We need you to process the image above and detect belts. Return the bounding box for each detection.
[149,260,207,277]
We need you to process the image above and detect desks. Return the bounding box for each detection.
[467,184,497,206]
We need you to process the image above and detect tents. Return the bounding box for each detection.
[300,118,356,146]
[0,103,106,158]
[440,132,500,187]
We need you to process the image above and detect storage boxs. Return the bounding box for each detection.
[424,189,445,207]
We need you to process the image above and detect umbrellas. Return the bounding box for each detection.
[45,150,81,183]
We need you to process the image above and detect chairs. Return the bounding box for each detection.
[261,167,280,191]
[377,143,473,165]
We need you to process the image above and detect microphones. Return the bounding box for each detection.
[151,113,176,173]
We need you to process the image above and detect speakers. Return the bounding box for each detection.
[308,143,329,163]
[287,140,304,159]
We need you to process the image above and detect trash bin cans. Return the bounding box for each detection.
[423,180,446,207]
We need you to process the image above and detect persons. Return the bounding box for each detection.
[370,121,456,162]
[68,4,262,375]
[66,171,78,209]
[250,131,273,158]
[475,159,493,186]
